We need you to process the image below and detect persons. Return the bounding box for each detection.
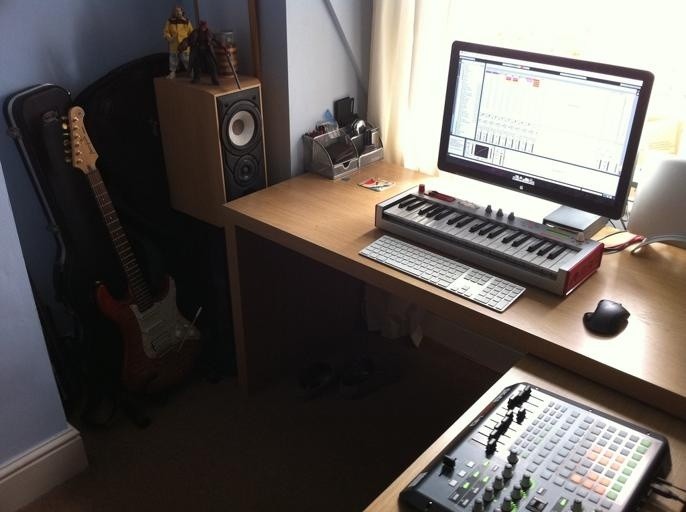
[175,20,232,86]
[162,3,195,80]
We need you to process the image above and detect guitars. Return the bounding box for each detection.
[60,105,209,393]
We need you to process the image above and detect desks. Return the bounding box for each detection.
[221,161,686,422]
[361,353,686,512]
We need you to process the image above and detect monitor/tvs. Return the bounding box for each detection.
[437,40,655,220]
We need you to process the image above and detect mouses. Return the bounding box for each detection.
[582,299,631,336]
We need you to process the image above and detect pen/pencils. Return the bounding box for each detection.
[306,126,326,140]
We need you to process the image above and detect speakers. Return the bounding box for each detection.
[152,73,269,228]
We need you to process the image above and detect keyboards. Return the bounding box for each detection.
[359,235,526,313]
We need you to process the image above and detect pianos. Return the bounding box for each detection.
[375,186,604,297]
